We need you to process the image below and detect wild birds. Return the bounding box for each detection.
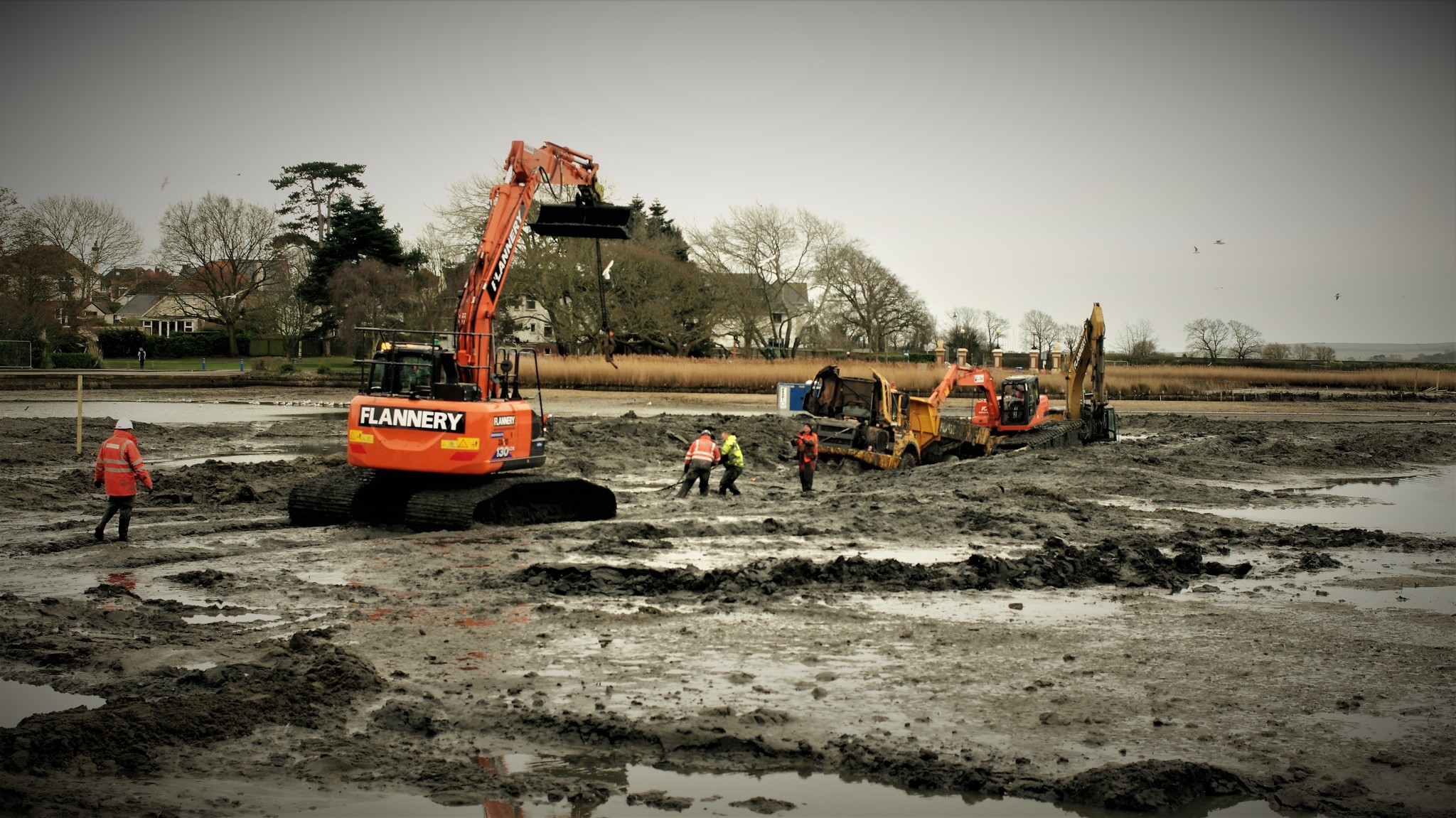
[1193,240,1226,253]
[1335,293,1341,301]
[602,259,614,279]
[216,288,249,299]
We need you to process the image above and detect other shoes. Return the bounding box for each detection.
[118,535,135,542]
[95,524,105,542]
[718,486,816,496]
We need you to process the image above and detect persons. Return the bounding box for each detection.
[93,418,153,541]
[1006,390,1024,423]
[791,423,818,492]
[138,348,146,369]
[675,429,721,498]
[718,430,744,495]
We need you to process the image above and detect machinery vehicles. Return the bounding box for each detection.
[795,361,944,475]
[913,364,1079,461]
[1042,301,1118,446]
[279,136,631,529]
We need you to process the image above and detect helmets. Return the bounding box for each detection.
[701,429,711,436]
[115,417,133,430]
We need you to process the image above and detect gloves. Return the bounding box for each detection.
[94,480,105,489]
[147,486,153,495]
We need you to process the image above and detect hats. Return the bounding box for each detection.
[804,423,813,430]
